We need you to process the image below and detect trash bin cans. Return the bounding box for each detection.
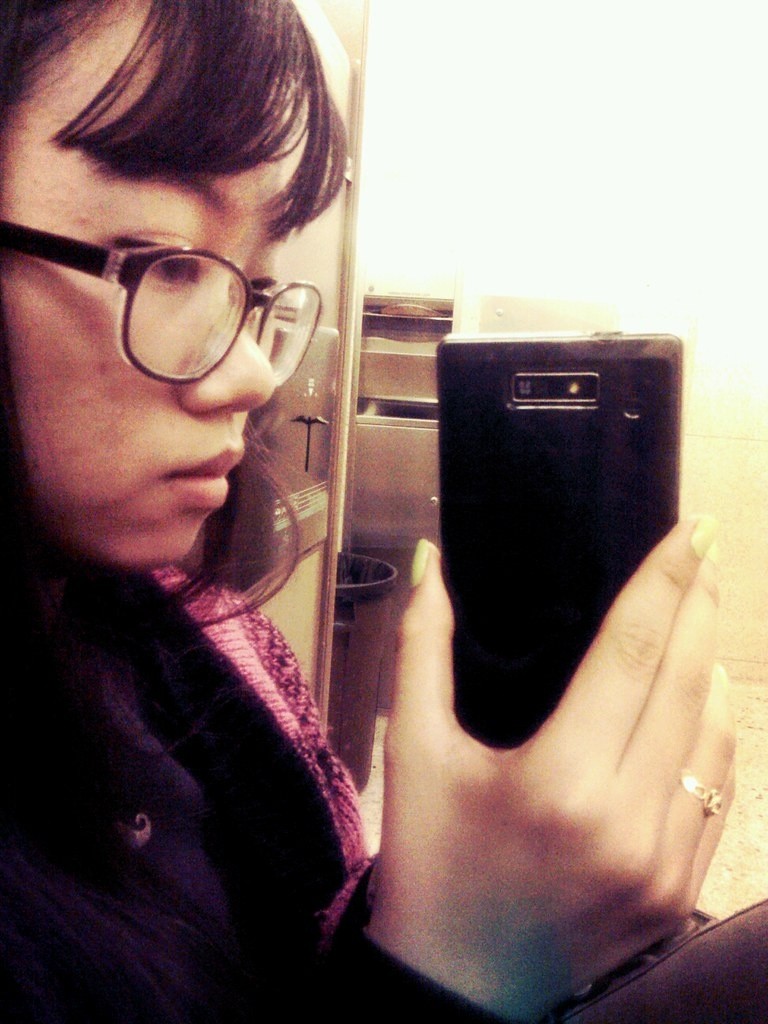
[326,550,399,795]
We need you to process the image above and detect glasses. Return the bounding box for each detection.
[0,216,328,390]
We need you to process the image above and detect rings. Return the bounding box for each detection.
[679,770,724,817]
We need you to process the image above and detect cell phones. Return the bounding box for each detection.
[434,331,685,748]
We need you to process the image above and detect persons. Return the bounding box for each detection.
[2,0,768,1024]
